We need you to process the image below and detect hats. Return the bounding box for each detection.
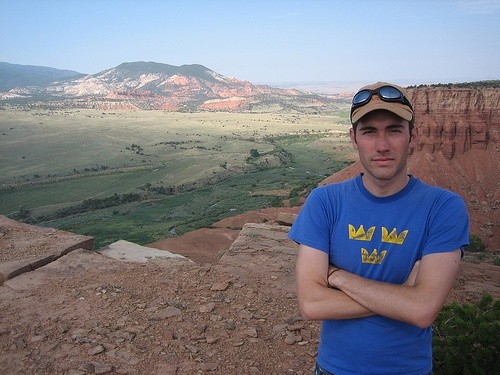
[348,81,415,123]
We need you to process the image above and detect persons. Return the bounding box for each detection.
[287,81,470,375]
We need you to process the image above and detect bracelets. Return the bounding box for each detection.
[326,267,344,288]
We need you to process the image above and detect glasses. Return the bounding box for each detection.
[350,85,412,109]
